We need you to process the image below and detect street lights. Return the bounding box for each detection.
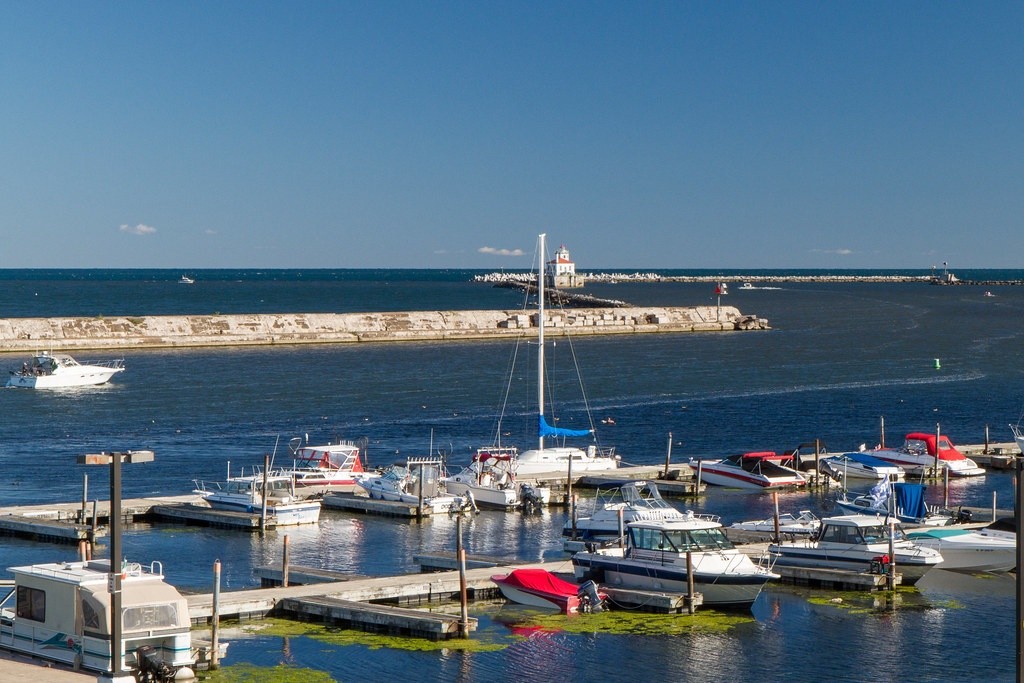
[80,450,155,679]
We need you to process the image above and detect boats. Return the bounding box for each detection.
[0,558,196,683]
[983,291,993,297]
[784,443,845,491]
[283,433,380,484]
[858,434,985,479]
[572,506,783,610]
[766,512,943,583]
[488,565,611,615]
[562,480,722,543]
[352,465,481,515]
[8,349,127,391]
[691,449,807,490]
[722,509,823,541]
[836,473,952,526]
[439,445,550,514]
[193,458,321,525]
[720,281,752,294]
[903,517,1018,577]
[823,450,904,479]
[1009,422,1024,453]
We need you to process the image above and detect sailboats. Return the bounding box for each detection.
[486,233,621,476]
